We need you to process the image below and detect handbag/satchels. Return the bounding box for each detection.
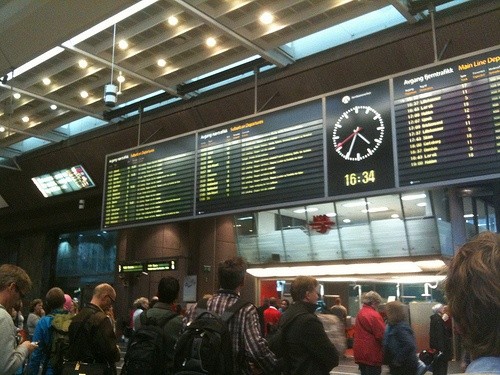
[57,361,110,375]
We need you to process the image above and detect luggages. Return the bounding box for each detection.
[415,348,445,375]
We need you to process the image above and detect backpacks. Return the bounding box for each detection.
[173,296,251,375]
[261,307,318,375]
[45,312,77,369]
[119,308,180,375]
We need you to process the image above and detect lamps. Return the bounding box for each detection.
[104,23,118,106]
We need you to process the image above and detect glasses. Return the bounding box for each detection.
[108,295,116,305]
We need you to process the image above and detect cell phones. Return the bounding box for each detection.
[31,341,38,345]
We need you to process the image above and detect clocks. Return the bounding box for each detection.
[332,106,384,161]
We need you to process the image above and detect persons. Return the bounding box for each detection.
[67,283,120,375]
[257,297,289,335]
[134,277,188,340]
[190,256,278,375]
[0,264,78,375]
[384,302,418,375]
[272,276,338,375]
[430,304,451,356]
[443,232,500,374]
[331,298,347,325]
[129,296,159,334]
[353,291,385,375]
